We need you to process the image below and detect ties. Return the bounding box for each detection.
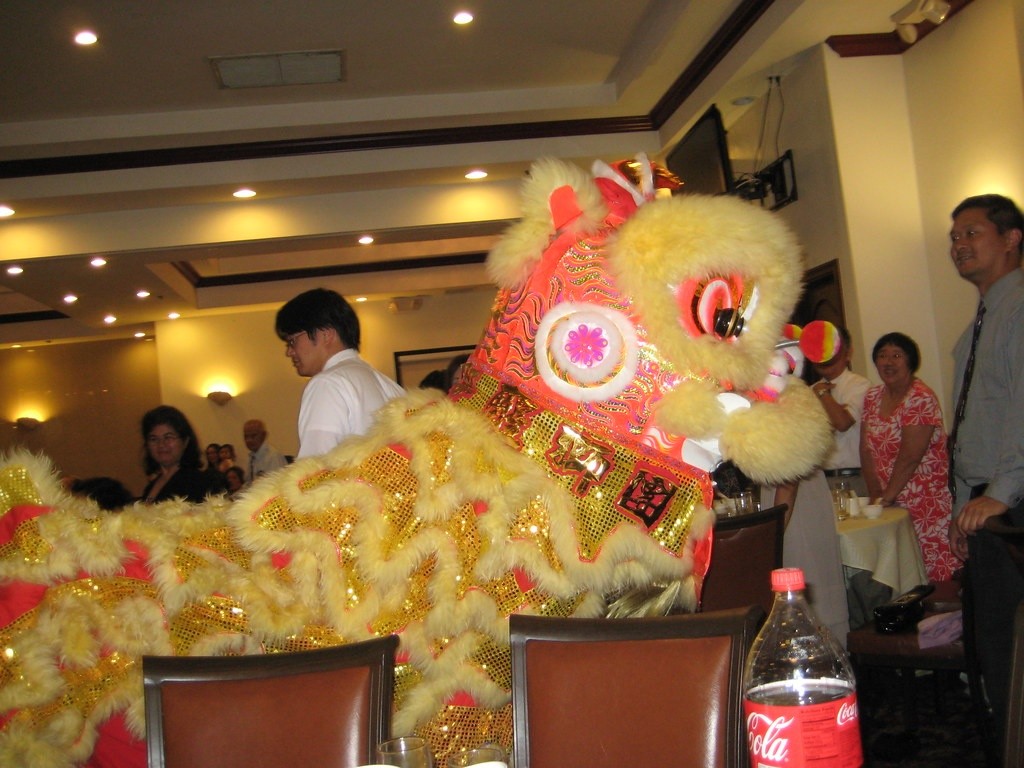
[949,301,987,503]
[250,456,254,481]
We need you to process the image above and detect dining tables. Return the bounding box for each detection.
[709,497,911,625]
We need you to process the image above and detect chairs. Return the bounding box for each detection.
[847,484,1000,709]
[142,635,399,768]
[510,604,764,768]
[858,598,1024,768]
[701,503,789,611]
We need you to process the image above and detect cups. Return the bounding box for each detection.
[846,498,859,518]
[374,736,433,768]
[447,744,508,768]
[734,491,754,515]
[831,495,847,521]
[355,764,400,768]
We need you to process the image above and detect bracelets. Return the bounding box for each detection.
[816,388,827,395]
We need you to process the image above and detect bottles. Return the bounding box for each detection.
[832,488,841,514]
[740,567,864,768]
[838,482,848,510]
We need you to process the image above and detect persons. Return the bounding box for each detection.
[60,408,294,511]
[805,329,872,503]
[859,332,968,606]
[273,286,406,457]
[947,194,1024,768]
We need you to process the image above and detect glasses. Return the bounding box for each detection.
[148,434,182,446]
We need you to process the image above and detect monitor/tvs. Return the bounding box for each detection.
[664,103,735,195]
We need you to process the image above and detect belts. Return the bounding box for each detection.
[825,468,858,477]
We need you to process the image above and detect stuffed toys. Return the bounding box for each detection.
[0,155,843,768]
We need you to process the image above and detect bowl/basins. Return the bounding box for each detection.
[712,498,736,515]
[862,504,883,520]
[858,496,870,508]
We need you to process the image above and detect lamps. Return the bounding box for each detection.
[208,391,232,406]
[17,418,41,431]
